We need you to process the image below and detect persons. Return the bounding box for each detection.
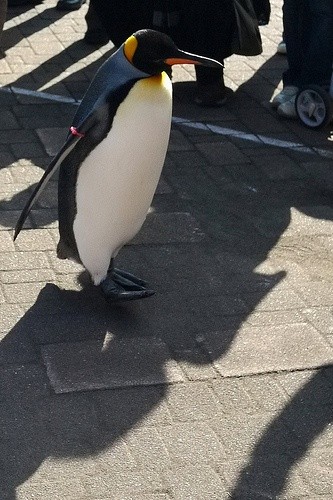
[6,0,85,12]
[276,0,288,54]
[81,0,271,106]
[271,0,332,121]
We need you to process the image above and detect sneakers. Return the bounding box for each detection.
[272,86,299,110]
[277,95,298,119]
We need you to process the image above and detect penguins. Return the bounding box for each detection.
[14,29,226,299]
